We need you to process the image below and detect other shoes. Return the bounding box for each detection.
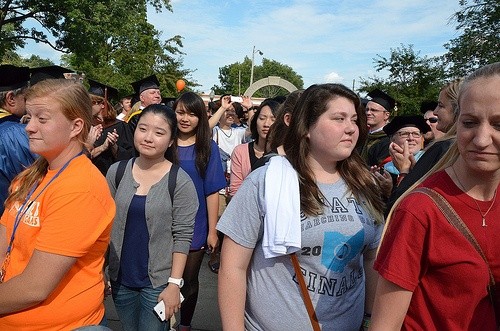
[177,323,191,331]
[208,252,220,274]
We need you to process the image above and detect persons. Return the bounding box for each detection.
[106,103,200,331]
[214,83,387,330]
[0,62,500,331]
[366,62,500,330]
[0,78,117,331]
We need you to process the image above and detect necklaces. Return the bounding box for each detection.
[451,164,498,227]
[219,127,232,137]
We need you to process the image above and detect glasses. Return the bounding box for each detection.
[91,100,103,106]
[225,109,237,114]
[392,131,422,138]
[424,117,439,123]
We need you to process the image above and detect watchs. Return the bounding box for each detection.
[396,172,408,182]
[168,277,184,289]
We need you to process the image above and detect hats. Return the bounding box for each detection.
[87,78,122,117]
[364,89,402,113]
[160,97,178,107]
[30,65,75,86]
[383,113,431,134]
[0,64,30,91]
[420,100,438,114]
[129,74,160,101]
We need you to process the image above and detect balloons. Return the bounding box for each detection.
[176,79,185,92]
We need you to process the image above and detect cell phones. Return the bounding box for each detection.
[153,291,186,321]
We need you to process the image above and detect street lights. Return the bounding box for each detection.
[250,45,264,88]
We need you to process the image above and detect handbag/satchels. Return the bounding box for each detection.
[215,128,230,174]
